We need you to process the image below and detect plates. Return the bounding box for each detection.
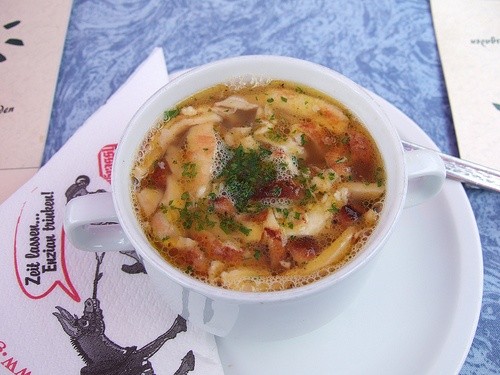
[167,70,485,374]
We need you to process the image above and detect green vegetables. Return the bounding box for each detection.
[159,85,386,260]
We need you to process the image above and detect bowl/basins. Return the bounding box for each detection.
[62,53,459,307]
